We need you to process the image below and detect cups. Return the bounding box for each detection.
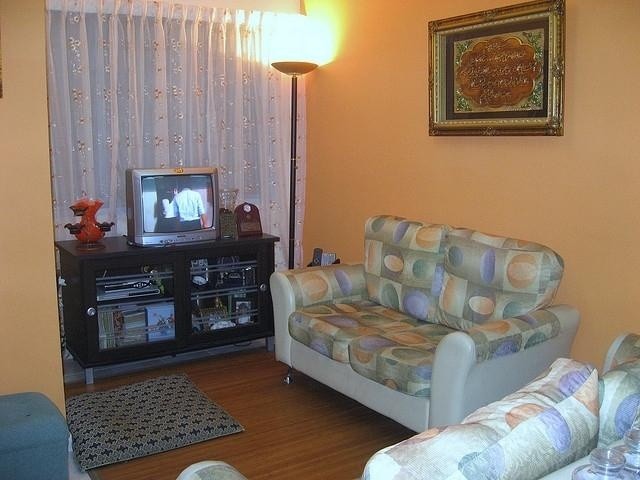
[219,188,239,214]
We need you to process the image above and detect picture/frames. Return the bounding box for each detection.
[426,0,565,137]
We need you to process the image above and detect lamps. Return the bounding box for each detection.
[271,57,318,269]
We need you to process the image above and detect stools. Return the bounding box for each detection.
[0,392,88,480]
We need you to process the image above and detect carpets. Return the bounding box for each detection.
[67,371,244,471]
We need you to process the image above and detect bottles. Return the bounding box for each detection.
[571,448,628,480]
[612,430,640,474]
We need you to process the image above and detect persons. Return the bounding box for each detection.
[172,180,211,230]
[236,303,251,325]
[155,182,176,231]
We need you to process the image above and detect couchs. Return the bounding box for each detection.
[175,335,640,480]
[271,215,580,436]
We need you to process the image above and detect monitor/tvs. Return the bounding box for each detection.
[125,166,221,247]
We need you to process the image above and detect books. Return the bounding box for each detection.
[98,301,176,349]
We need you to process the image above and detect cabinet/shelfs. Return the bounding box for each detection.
[56,227,282,384]
